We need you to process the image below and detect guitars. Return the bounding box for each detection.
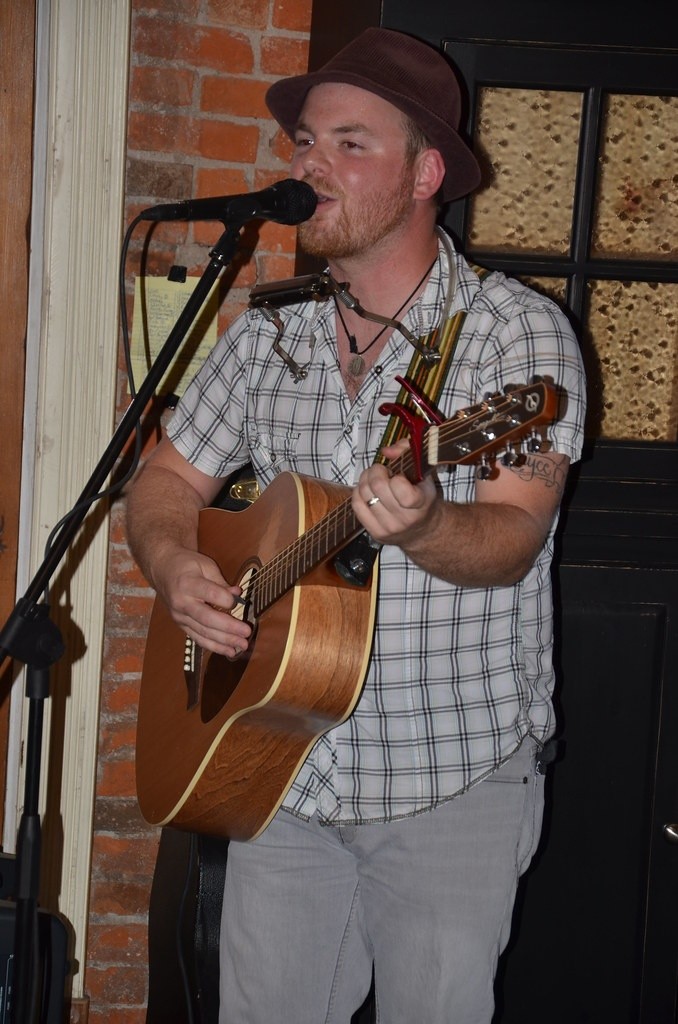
[134,374,558,843]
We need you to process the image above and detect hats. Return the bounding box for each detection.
[264,27,482,206]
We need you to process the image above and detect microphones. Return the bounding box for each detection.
[141,178,318,226]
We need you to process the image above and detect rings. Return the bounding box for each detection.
[367,497,380,507]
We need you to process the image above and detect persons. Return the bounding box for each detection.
[126,26,587,1024]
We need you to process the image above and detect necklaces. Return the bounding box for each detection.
[333,257,437,378]
